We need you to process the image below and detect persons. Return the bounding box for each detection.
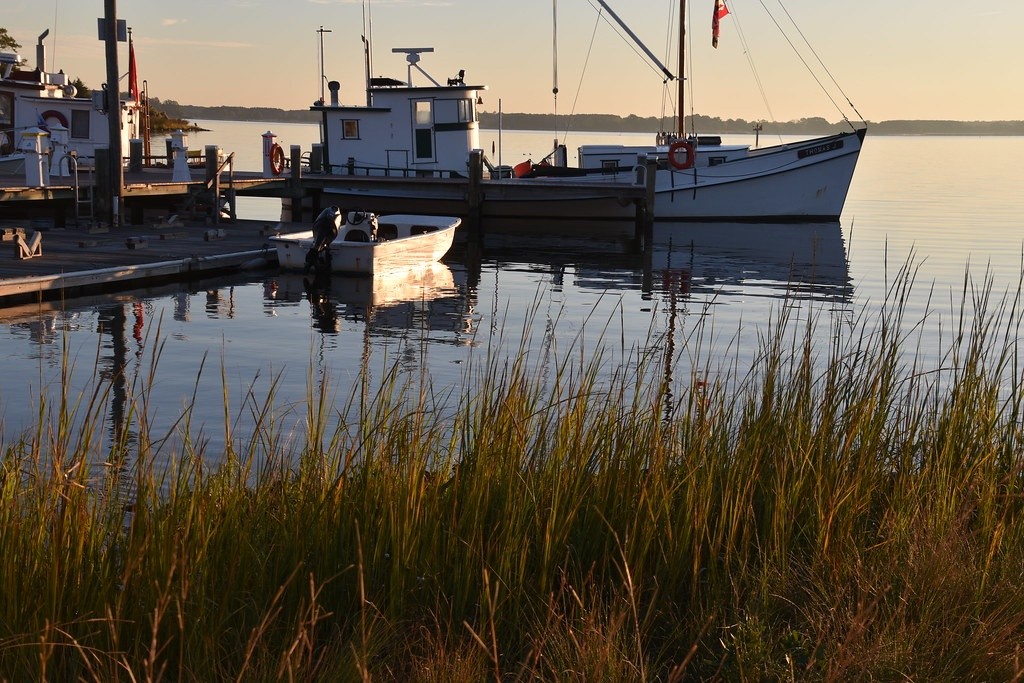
[313,205,338,239]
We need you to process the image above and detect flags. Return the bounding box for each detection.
[712,0,731,49]
[129,44,138,108]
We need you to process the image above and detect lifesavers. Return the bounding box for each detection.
[270,143,285,175]
[38,110,68,137]
[668,142,694,170]
[664,270,689,295]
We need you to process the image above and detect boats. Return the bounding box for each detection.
[269,211,461,273]
[280,0,867,222]
[0,21,151,186]
